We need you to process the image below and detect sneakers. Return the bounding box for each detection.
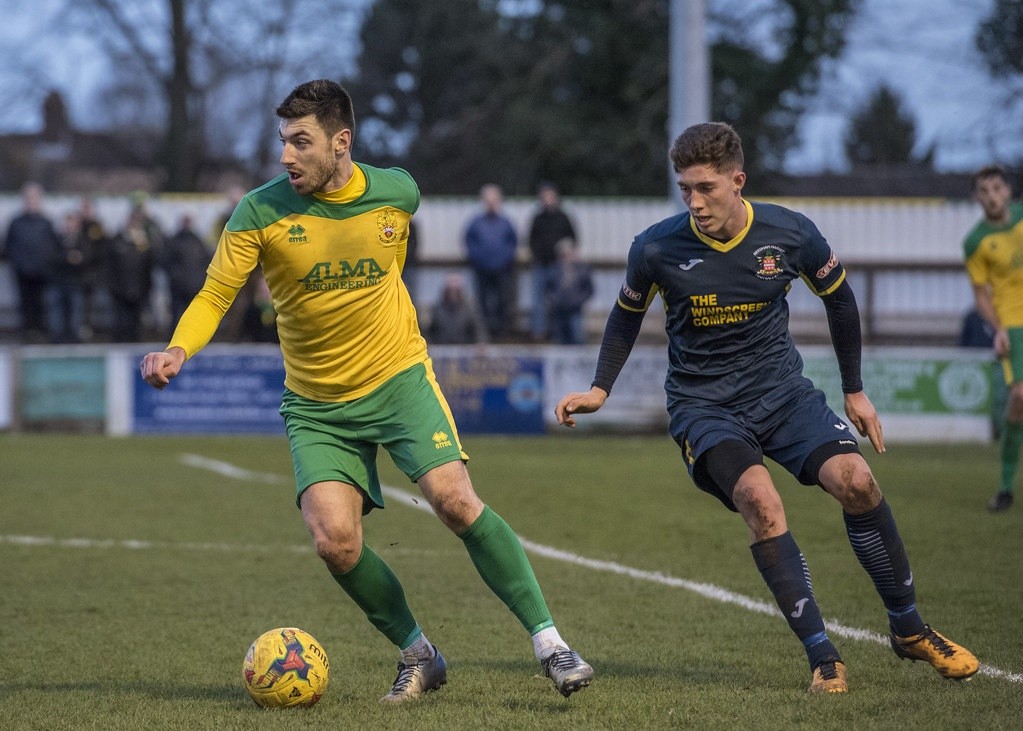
[540,645,595,697]
[887,621,980,679]
[986,488,1014,512]
[809,652,850,696]
[378,644,447,705]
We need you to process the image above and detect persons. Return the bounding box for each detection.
[540,236,595,344]
[529,188,575,337]
[427,282,475,346]
[147,78,594,702]
[0,183,279,345]
[553,122,979,691]
[962,164,1023,514]
[466,186,520,344]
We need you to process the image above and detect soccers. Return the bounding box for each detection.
[243,627,328,707]
